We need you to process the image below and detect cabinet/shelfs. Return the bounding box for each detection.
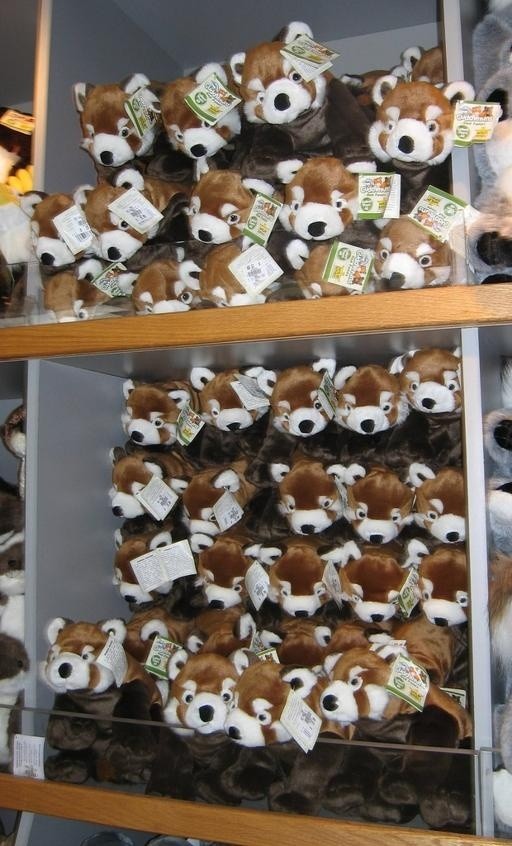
[1,1,511,846]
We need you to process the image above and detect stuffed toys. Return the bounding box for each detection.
[2,0,512,322]
[2,2,512,846]
[0,394,27,844]
[39,346,512,846]
